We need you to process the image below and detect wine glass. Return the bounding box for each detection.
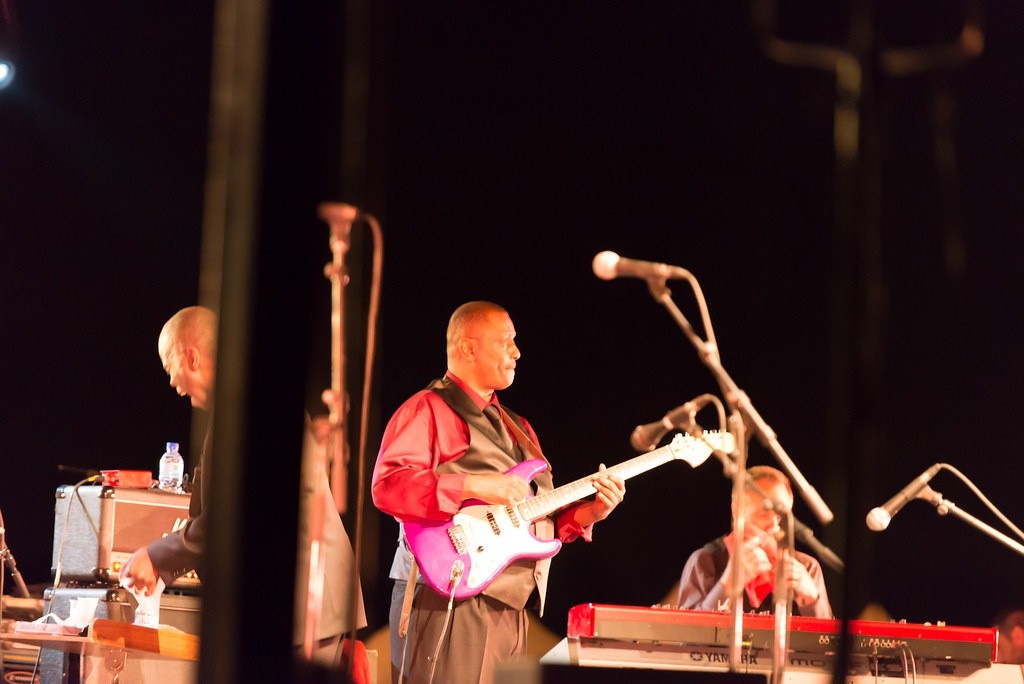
[104,649,128,684]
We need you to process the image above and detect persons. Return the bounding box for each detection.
[371,300,626,683]
[122,305,367,667]
[675,466,834,619]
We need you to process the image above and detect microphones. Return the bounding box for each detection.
[594,251,688,280]
[866,464,941,531]
[631,395,712,451]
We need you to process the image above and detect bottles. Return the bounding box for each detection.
[159,442,184,492]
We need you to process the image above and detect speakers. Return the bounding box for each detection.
[39,587,203,684]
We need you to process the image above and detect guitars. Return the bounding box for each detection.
[401,428,735,602]
